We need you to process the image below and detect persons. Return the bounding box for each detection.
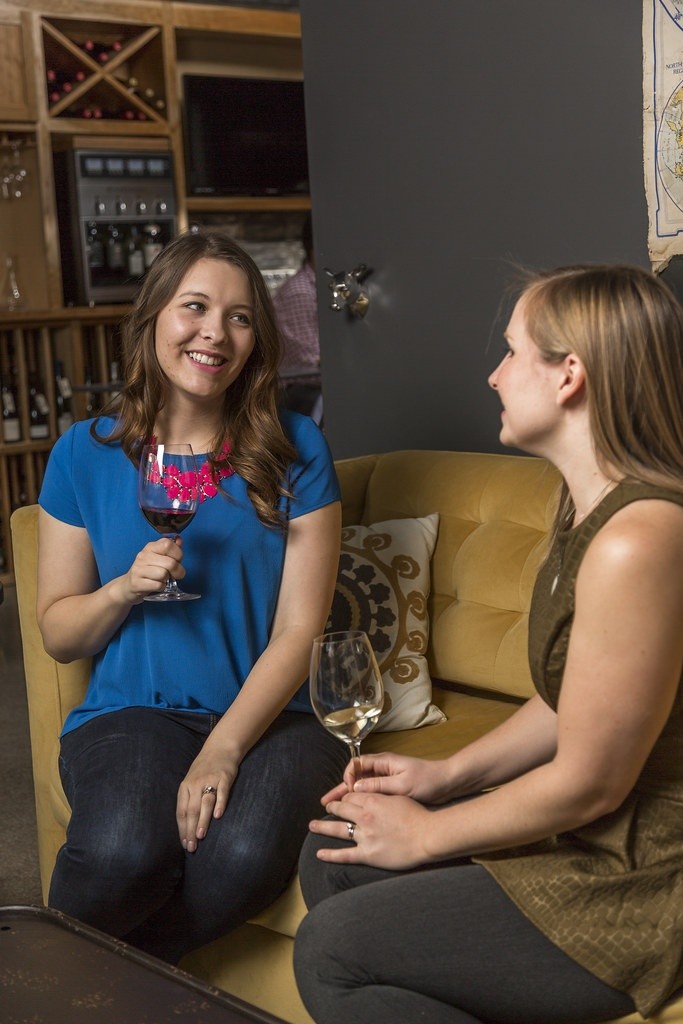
[293,267,682,1024]
[37,230,351,966]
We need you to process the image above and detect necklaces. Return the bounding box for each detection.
[551,472,618,595]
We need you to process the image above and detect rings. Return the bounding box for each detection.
[347,823,356,839]
[202,786,217,796]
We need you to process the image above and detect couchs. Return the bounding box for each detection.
[11,451,683,1024]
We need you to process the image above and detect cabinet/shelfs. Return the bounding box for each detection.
[0,0,321,422]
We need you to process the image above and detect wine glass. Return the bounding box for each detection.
[308,627,384,791]
[139,440,204,603]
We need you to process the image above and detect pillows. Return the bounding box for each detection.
[313,512,447,735]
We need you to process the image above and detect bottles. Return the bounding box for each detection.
[1,349,126,444]
[45,38,167,122]
[88,223,172,288]
